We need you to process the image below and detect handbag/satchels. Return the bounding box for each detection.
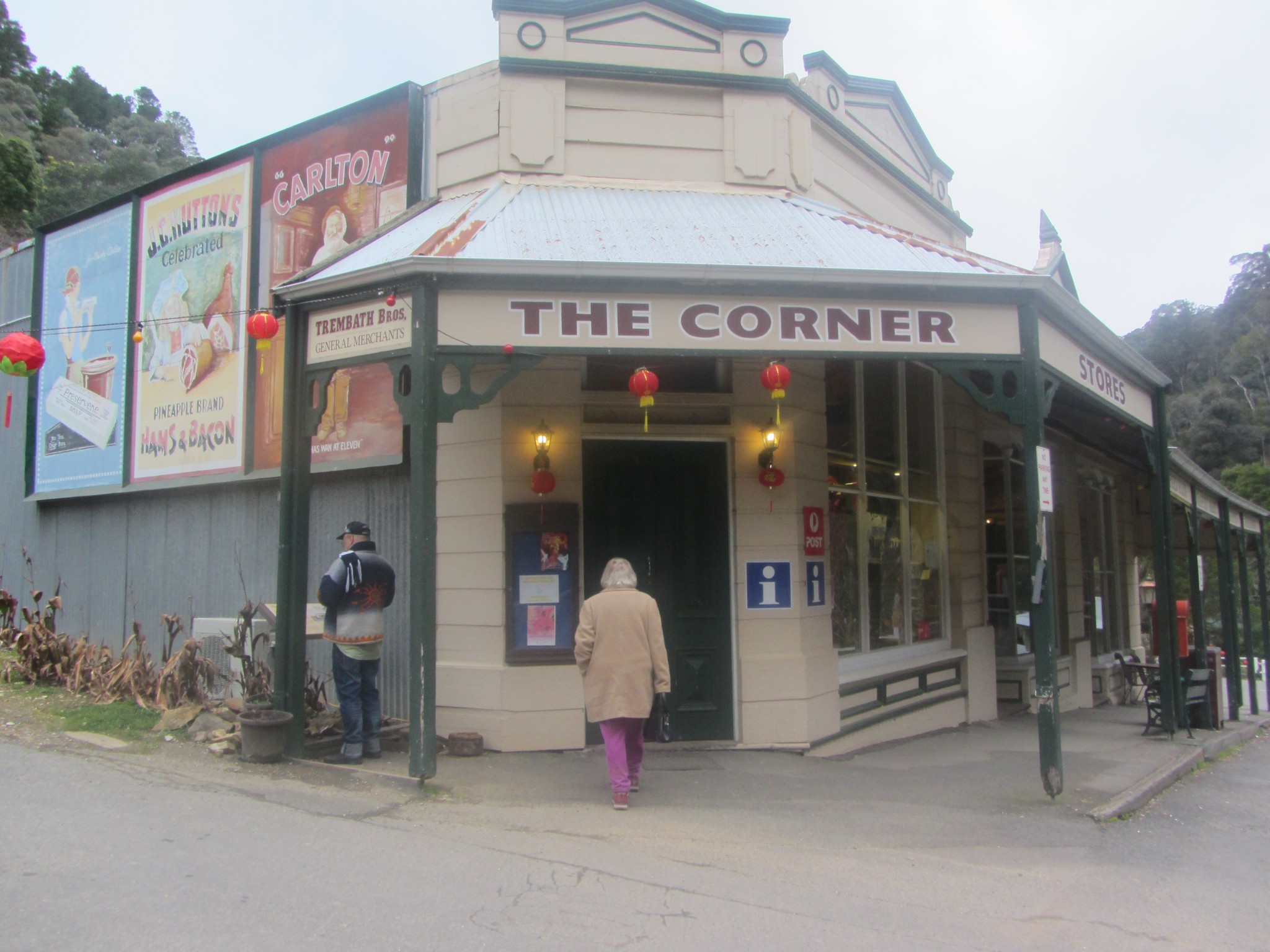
[643,692,673,742]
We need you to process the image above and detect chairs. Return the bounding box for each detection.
[1113,649,1215,740]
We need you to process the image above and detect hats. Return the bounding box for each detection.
[336,521,370,540]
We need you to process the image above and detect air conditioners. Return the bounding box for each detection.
[189,618,269,702]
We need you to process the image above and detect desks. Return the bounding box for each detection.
[1124,662,1160,702]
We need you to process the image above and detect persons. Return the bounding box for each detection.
[573,557,671,810]
[318,523,396,765]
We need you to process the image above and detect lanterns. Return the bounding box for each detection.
[530,466,556,524]
[759,460,785,513]
[0,331,46,428]
[248,310,279,375]
[761,361,791,427]
[628,366,660,432]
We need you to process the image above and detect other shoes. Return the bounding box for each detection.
[323,752,363,765]
[362,751,381,758]
[612,792,629,810]
[628,775,639,792]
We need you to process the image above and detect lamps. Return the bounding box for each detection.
[758,417,782,468]
[531,417,553,470]
